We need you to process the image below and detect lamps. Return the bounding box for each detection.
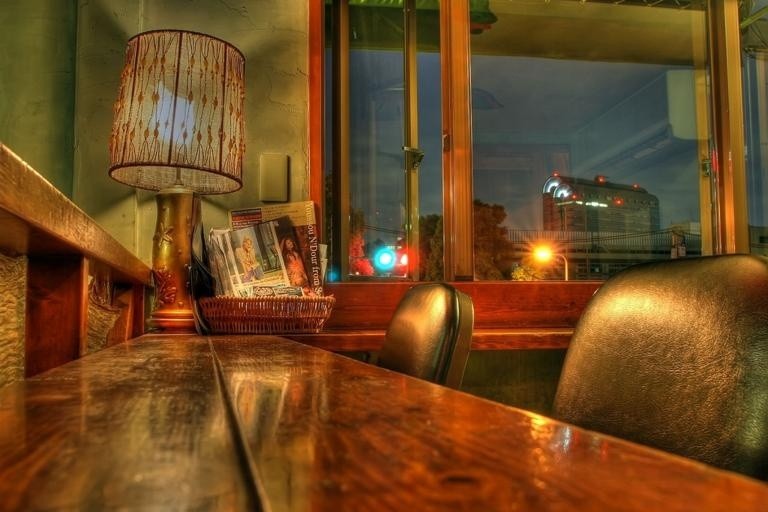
[108,28,245,330]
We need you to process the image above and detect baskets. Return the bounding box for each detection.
[199,295,336,334]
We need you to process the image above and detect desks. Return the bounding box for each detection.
[3,328,768,511]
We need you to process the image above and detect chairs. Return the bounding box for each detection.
[550,254,768,481]
[376,282,475,390]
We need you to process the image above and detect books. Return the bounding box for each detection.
[207,197,328,299]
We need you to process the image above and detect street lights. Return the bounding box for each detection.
[529,244,568,281]
